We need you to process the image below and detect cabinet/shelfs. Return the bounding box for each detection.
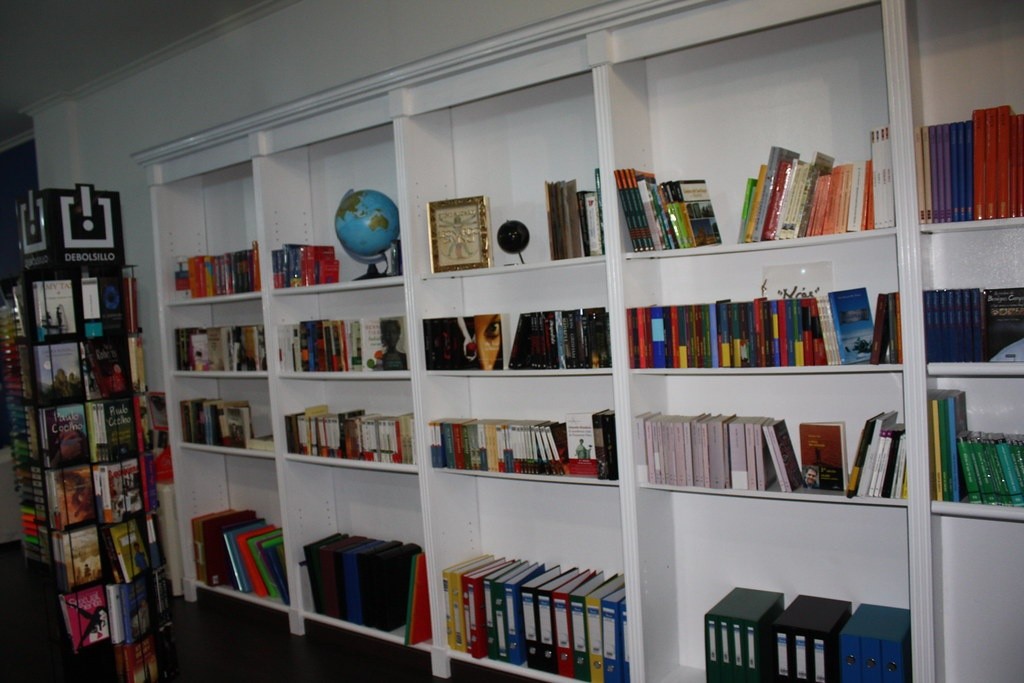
[0,183,181,683]
[127,0,1024,683]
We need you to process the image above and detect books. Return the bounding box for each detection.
[930,391,1023,509]
[443,555,628,682]
[422,306,611,371]
[277,319,409,372]
[284,406,415,464]
[172,241,262,299]
[634,411,906,498]
[180,400,274,449]
[429,408,617,480]
[917,107,1023,223]
[172,325,266,372]
[627,288,902,369]
[271,243,339,288]
[925,288,1024,365]
[189,510,289,604]
[303,532,431,644]
[614,127,896,252]
[543,168,605,259]
[0,273,173,683]
[705,587,911,683]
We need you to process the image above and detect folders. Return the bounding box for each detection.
[442,554,630,683]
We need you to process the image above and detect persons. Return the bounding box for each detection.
[801,465,820,491]
[474,316,503,370]
[376,318,408,370]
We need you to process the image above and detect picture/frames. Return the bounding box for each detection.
[427,195,494,273]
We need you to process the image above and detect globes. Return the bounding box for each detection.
[334,189,400,281]
